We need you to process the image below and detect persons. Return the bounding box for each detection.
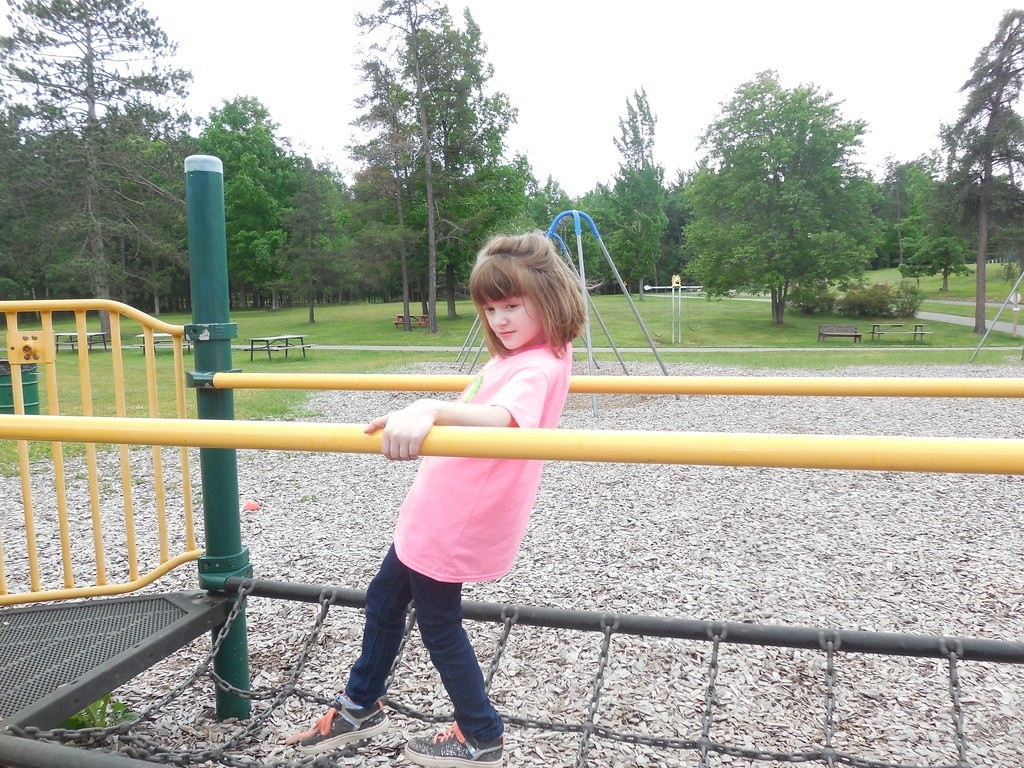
[298,229,603,768]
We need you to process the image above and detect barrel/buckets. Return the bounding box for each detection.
[0,358,40,415]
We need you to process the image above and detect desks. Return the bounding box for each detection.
[394,314,428,328]
[135,333,193,355]
[54,332,109,353]
[867,323,929,342]
[244,335,308,363]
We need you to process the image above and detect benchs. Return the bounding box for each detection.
[818,324,862,344]
[55,339,112,353]
[244,345,311,363]
[140,340,193,355]
[394,319,428,328]
[865,329,933,342]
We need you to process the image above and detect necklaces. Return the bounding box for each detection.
[454,351,512,404]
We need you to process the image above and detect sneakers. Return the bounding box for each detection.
[298,690,390,755]
[403,721,506,768]
